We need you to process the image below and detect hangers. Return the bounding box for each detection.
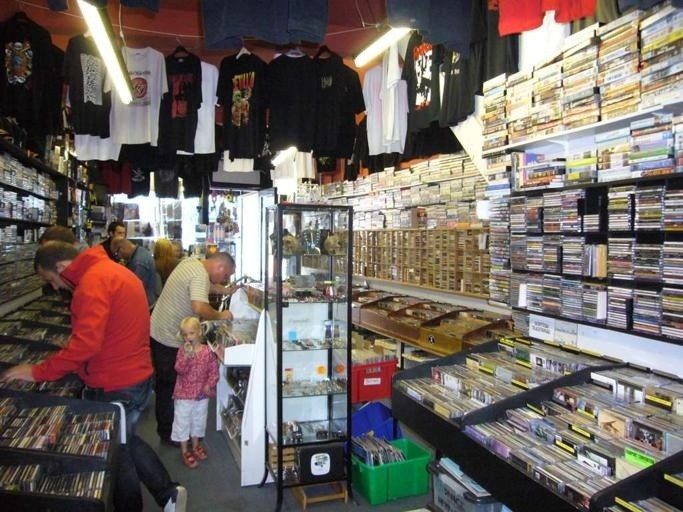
[13,2,28,23]
[172,37,192,60]
[83,29,92,39]
[235,36,252,60]
[284,44,334,61]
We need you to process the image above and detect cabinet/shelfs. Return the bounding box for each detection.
[215,99,683,511]
[1,388,122,463]
[1,127,89,398]
[88,184,214,254]
[1,448,118,510]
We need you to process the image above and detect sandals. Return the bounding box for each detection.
[181,445,209,468]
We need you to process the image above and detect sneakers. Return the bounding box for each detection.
[162,484,187,512]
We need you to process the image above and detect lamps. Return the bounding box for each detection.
[79,1,136,109]
[353,21,415,69]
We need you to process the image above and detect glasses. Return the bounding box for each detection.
[111,246,121,258]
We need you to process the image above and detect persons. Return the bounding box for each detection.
[150,252,241,447]
[170,317,219,468]
[38,222,181,316]
[0,240,187,512]
